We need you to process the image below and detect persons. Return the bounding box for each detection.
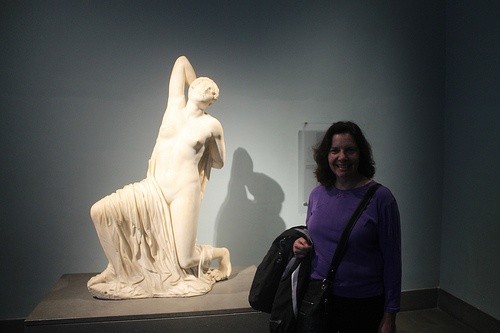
[293,122,401,333]
[86,56,233,289]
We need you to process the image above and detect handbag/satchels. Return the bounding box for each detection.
[297,279,331,333]
